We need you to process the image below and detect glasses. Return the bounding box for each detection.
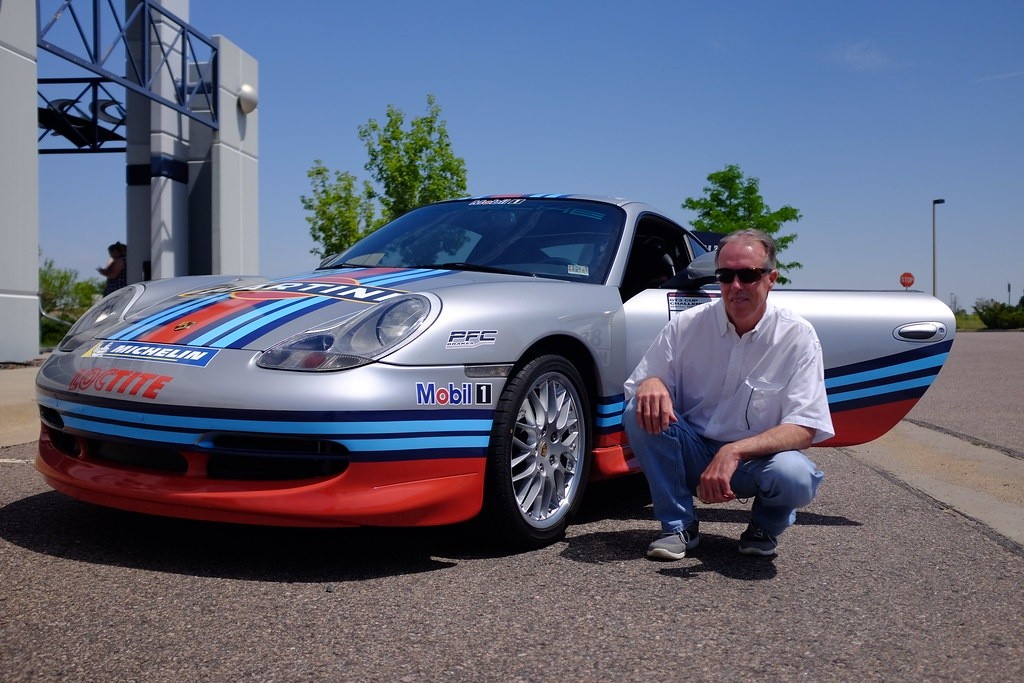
[715,266,771,283]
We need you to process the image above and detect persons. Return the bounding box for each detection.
[94,240,127,300]
[621,227,836,559]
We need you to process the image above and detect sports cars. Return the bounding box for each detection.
[35,192,957,551]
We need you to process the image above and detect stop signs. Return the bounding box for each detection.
[899,272,914,288]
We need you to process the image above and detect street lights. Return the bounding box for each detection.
[932,199,945,297]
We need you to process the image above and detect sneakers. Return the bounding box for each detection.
[647,522,701,560]
[737,522,778,555]
